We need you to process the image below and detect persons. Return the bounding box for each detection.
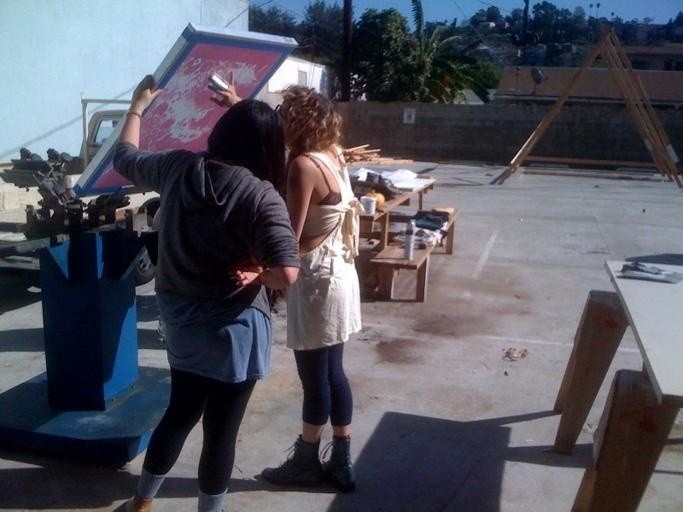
[207,70,365,494]
[114,74,300,512]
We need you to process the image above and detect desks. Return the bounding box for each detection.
[552,260,682,512]
[354,174,436,253]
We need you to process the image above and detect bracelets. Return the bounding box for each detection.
[126,112,142,120]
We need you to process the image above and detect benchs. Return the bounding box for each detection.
[372,205,462,302]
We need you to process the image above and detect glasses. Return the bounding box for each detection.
[274,104,289,123]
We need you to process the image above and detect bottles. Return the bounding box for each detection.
[405,220,415,262]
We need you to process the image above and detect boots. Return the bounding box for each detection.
[322,437,356,494]
[262,434,324,488]
